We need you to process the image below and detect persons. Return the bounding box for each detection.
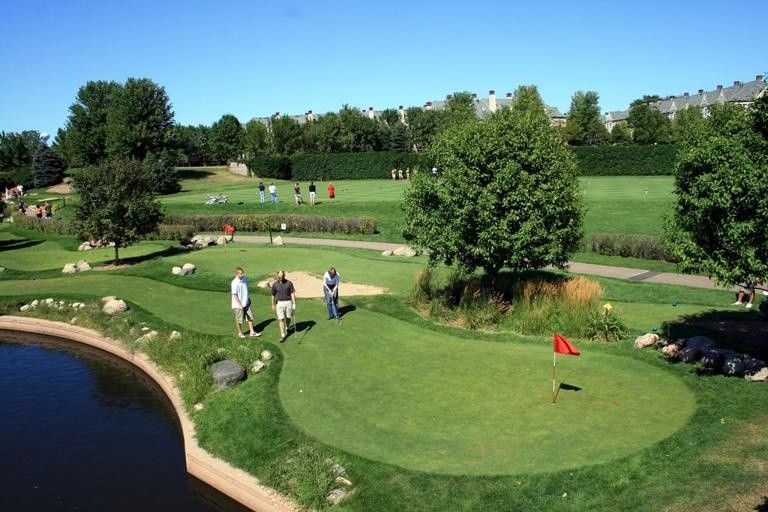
[391,167,396,180]
[268,182,277,204]
[406,167,409,179]
[399,168,403,180]
[432,166,437,176]
[5,183,52,220]
[328,183,336,205]
[322,268,341,321]
[734,279,755,308]
[270,271,296,343]
[294,182,301,206]
[259,182,265,203]
[309,181,317,206]
[229,267,263,337]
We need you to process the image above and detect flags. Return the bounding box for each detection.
[225,224,235,233]
[555,334,580,356]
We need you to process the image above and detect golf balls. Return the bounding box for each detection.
[300,389,303,392]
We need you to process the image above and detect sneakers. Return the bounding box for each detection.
[250,333,261,336]
[239,333,246,338]
[280,334,287,342]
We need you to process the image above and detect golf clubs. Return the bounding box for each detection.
[293,310,298,340]
